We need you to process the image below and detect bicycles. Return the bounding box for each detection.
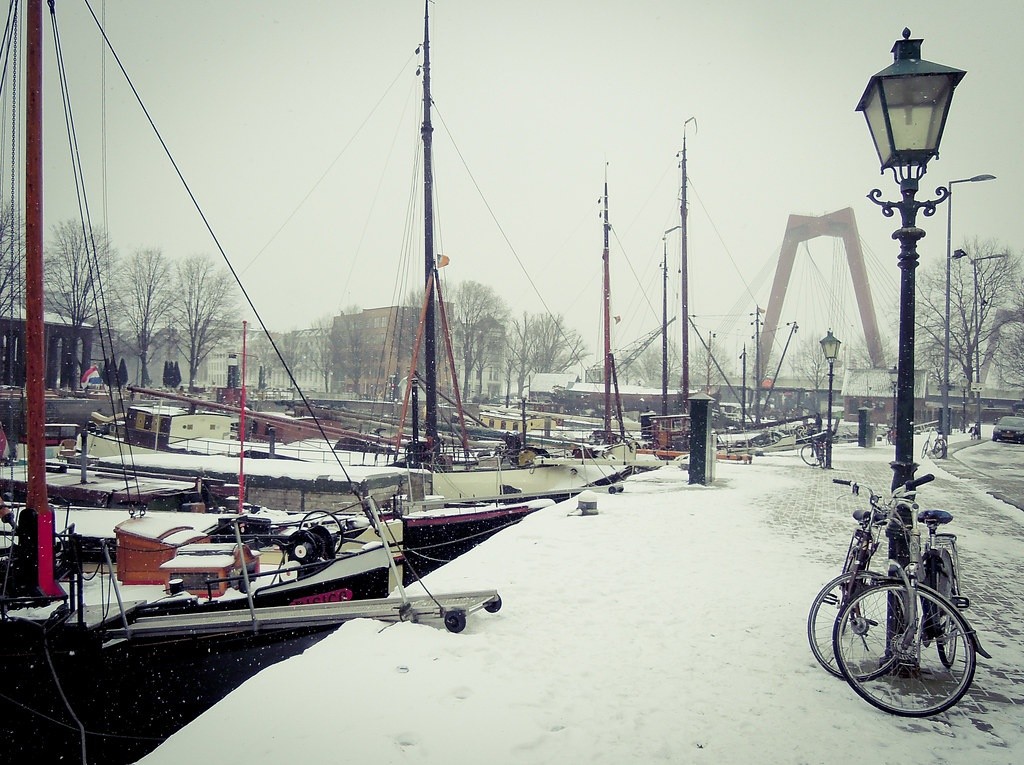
[801,426,826,469]
[885,426,897,446]
[810,473,994,719]
[921,427,948,459]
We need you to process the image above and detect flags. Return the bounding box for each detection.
[80,365,103,390]
[436,253,450,268]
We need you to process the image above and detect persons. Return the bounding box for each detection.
[815,412,822,432]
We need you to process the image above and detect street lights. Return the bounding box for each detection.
[852,24,967,679]
[941,173,997,438]
[661,225,684,417]
[973,253,1005,440]
[887,366,898,427]
[819,331,842,467]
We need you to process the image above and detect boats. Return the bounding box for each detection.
[0,0,637,765]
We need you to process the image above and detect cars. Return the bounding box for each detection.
[992,416,1024,445]
